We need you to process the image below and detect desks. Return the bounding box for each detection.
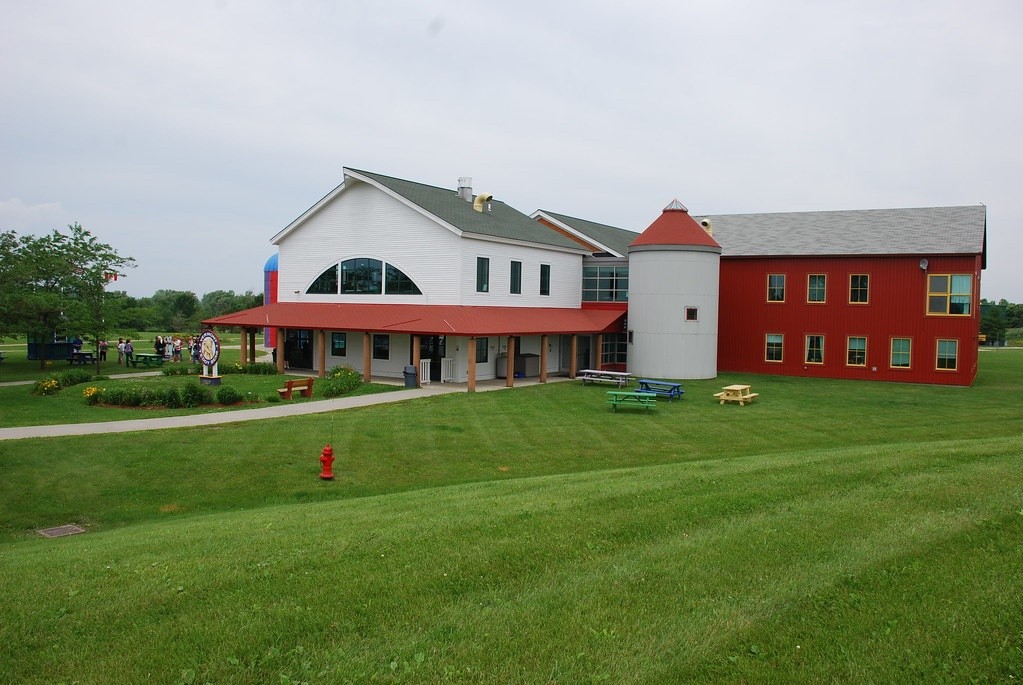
[135,353,163,367]
[607,390,656,414]
[638,379,683,402]
[580,369,633,387]
[720,383,751,405]
[70,351,95,364]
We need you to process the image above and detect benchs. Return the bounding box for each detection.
[577,375,636,387]
[66,356,98,364]
[634,386,684,403]
[130,359,169,367]
[276,376,315,399]
[606,398,656,414]
[712,391,759,405]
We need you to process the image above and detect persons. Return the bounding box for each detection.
[98,339,108,361]
[116,338,135,367]
[73,335,83,364]
[187,331,200,364]
[154,335,185,362]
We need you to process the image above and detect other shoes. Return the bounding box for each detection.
[177,360,182,363]
[169,360,174,363]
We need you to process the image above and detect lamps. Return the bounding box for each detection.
[919,258,929,272]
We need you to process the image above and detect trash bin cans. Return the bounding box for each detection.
[403,364,417,389]
